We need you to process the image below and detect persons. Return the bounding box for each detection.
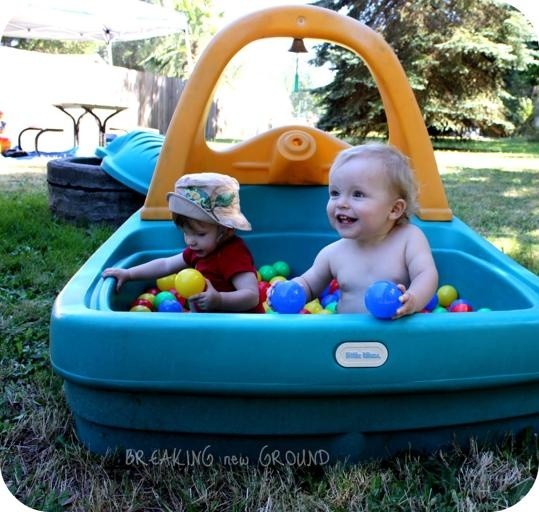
[100,171,266,312]
[264,140,439,320]
[130,261,495,313]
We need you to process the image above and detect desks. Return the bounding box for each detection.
[52,102,129,156]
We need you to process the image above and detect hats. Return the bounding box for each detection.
[165,172,253,232]
